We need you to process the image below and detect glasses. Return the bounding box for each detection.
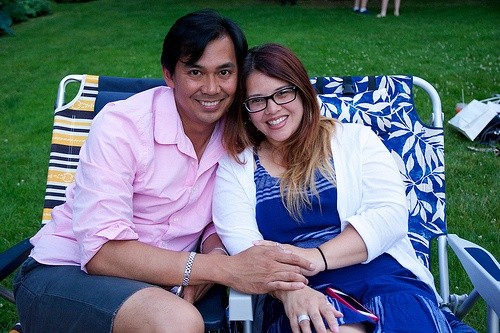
[242,86,298,113]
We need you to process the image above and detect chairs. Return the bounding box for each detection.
[0,75,500,333]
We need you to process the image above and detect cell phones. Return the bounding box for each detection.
[325,287,379,321]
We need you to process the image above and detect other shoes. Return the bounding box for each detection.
[354,10,359,14]
[361,11,368,14]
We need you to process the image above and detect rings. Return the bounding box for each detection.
[297,315,310,323]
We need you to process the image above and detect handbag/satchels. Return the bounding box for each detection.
[448,97,500,142]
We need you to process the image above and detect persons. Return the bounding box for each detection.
[353,0,370,15]
[212,42,451,333]
[377,0,401,17]
[13,7,249,333]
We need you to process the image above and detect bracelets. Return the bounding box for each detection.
[183,251,196,287]
[316,246,328,272]
[214,247,230,256]
